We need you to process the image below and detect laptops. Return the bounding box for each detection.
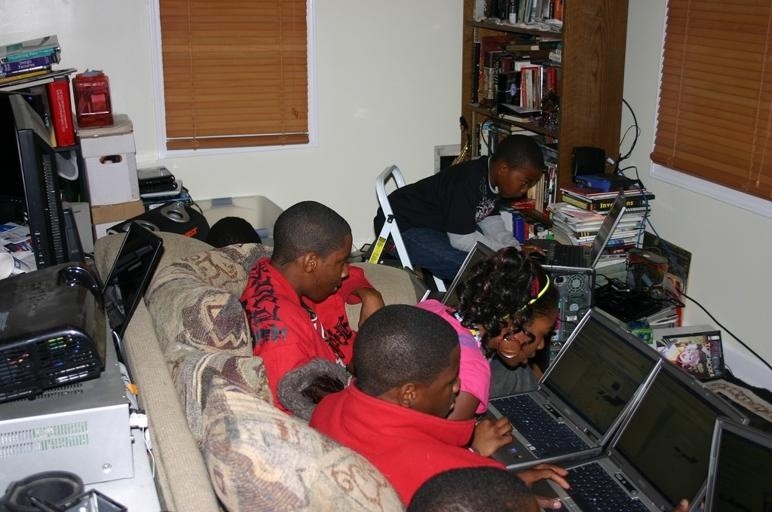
[470,308,665,474]
[704,415,772,511]
[530,358,749,512]
[441,241,496,314]
[523,187,626,269]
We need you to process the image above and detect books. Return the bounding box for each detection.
[493,159,656,260]
[0,34,63,84]
[632,305,726,383]
[470,0,565,159]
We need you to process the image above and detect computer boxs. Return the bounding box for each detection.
[548,272,595,352]
[0,309,134,498]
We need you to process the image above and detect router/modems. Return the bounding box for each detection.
[572,143,607,181]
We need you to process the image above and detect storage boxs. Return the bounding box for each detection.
[77,116,144,239]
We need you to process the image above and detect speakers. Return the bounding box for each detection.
[106,200,209,249]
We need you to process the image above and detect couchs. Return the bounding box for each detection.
[93,224,437,512]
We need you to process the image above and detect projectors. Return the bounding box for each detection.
[0,256,108,402]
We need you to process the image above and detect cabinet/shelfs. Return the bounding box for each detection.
[460,0,630,223]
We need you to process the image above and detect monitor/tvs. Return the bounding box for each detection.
[16,126,83,262]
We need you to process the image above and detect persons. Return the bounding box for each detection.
[408,245,562,461]
[406,467,543,512]
[240,201,386,418]
[307,304,706,512]
[374,133,551,284]
